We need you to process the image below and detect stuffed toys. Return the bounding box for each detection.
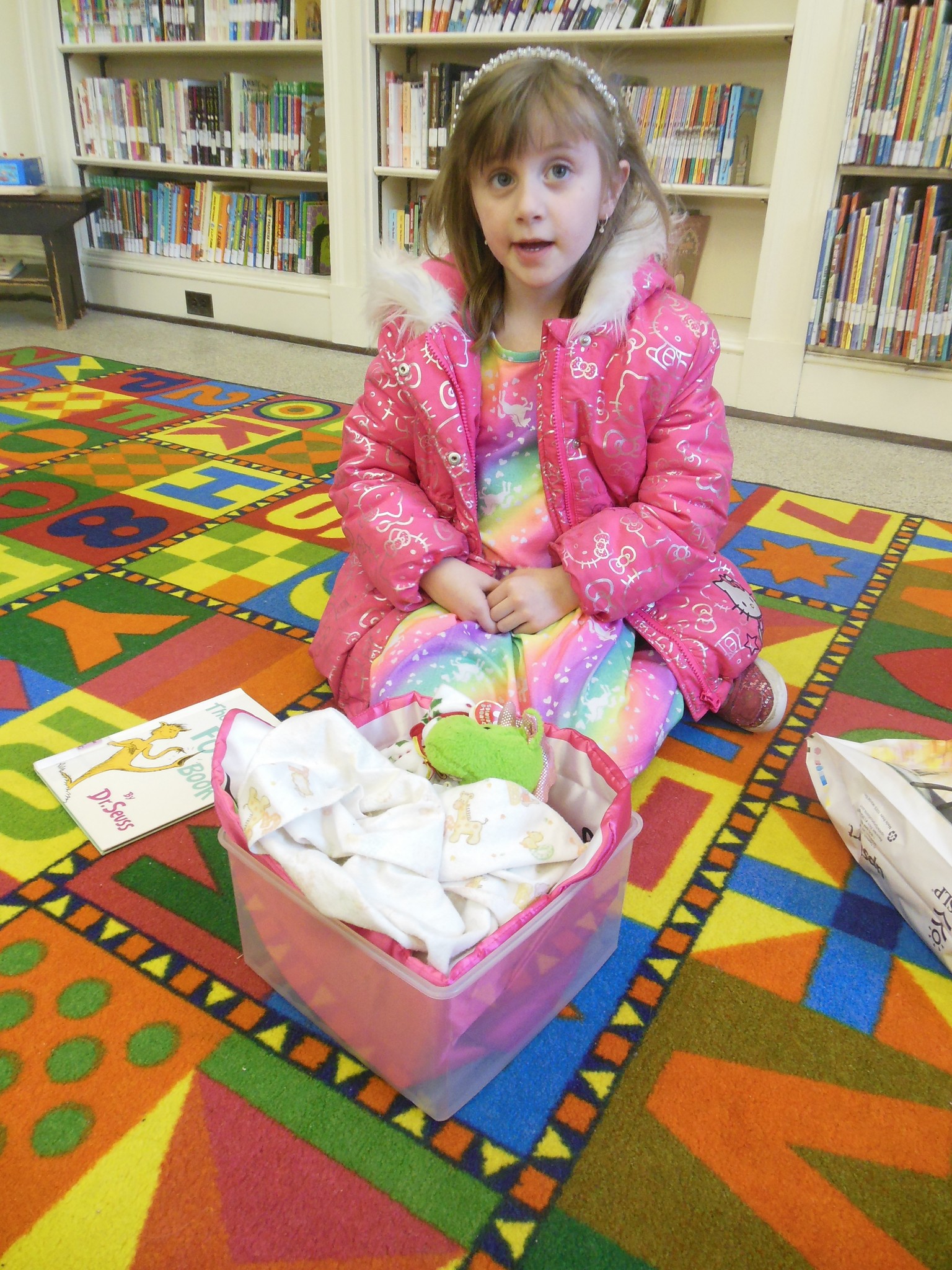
[415,701,547,800]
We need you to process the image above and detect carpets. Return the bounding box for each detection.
[0,338,952,1270]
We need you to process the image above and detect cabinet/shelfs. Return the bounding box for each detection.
[805,3,952,372]
[365,0,797,338]
[53,0,332,281]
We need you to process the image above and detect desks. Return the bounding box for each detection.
[0,186,108,331]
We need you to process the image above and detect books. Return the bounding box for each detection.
[57,0,330,280]
[0,257,23,276]
[802,1,952,372]
[376,0,766,303]
[0,263,26,281]
[26,687,281,857]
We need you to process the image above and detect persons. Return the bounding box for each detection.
[310,47,791,795]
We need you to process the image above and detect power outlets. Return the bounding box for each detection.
[185,290,215,318]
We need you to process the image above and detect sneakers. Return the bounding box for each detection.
[716,655,788,732]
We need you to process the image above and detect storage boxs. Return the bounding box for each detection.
[209,690,645,1121]
